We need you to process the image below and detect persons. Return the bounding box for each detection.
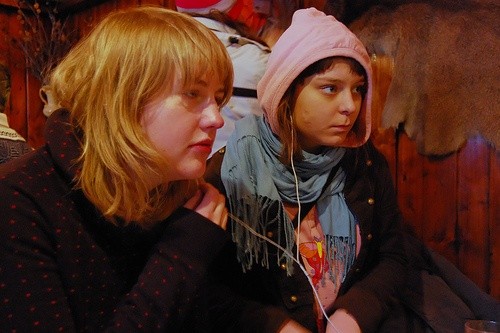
[0,7,310,333]
[204,6,427,333]
[175,0,285,162]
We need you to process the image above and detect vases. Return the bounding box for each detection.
[39,85,59,117]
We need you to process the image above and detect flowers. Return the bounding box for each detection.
[12,0,81,85]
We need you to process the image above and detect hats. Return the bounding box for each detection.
[175,0,235,14]
